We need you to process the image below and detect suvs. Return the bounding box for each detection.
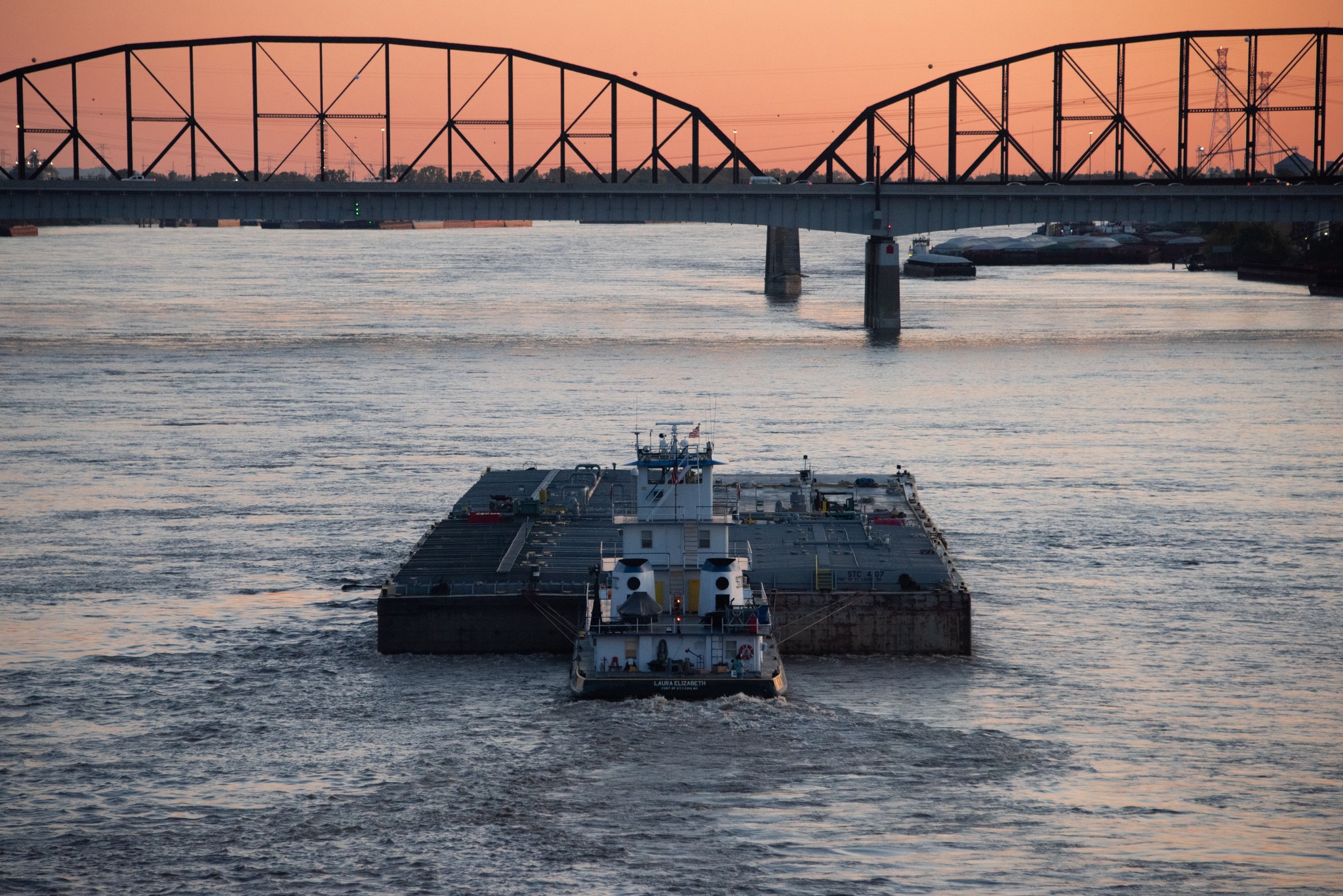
[788,180,812,185]
[1293,181,1318,186]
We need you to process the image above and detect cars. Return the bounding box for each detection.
[1044,182,1064,186]
[1333,182,1343,185]
[1166,182,1184,186]
[857,182,876,185]
[1133,182,1156,186]
[1004,182,1027,185]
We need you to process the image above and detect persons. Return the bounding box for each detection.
[733,653,744,679]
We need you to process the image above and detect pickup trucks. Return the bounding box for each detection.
[1247,178,1289,186]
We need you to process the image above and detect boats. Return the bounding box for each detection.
[567,420,789,702]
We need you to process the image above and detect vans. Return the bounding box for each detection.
[749,176,781,184]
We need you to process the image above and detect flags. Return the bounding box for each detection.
[689,426,699,438]
